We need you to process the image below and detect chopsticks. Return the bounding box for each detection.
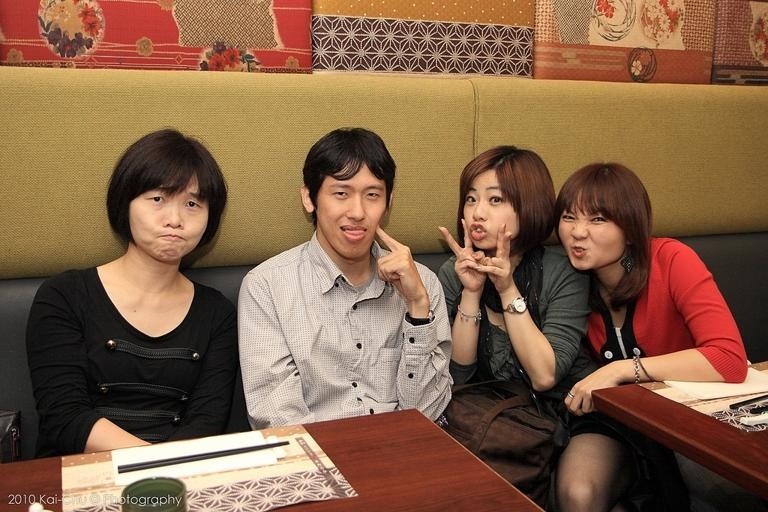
[118,440,289,474]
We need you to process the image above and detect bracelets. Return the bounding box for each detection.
[633,355,654,383]
[457,305,481,324]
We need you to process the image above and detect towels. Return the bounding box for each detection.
[636,361,768,432]
[61,424,359,512]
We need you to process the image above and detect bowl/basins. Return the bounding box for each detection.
[123,477,186,512]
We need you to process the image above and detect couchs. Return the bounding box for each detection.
[0,65,768,464]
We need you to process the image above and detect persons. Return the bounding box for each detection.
[25,130,238,460]
[434,145,598,428]
[232,127,454,430]
[555,162,747,512]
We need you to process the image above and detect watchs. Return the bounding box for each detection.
[405,311,436,326]
[503,296,527,313]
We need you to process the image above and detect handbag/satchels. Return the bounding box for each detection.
[442,391,555,483]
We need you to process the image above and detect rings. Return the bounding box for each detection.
[568,391,574,399]
